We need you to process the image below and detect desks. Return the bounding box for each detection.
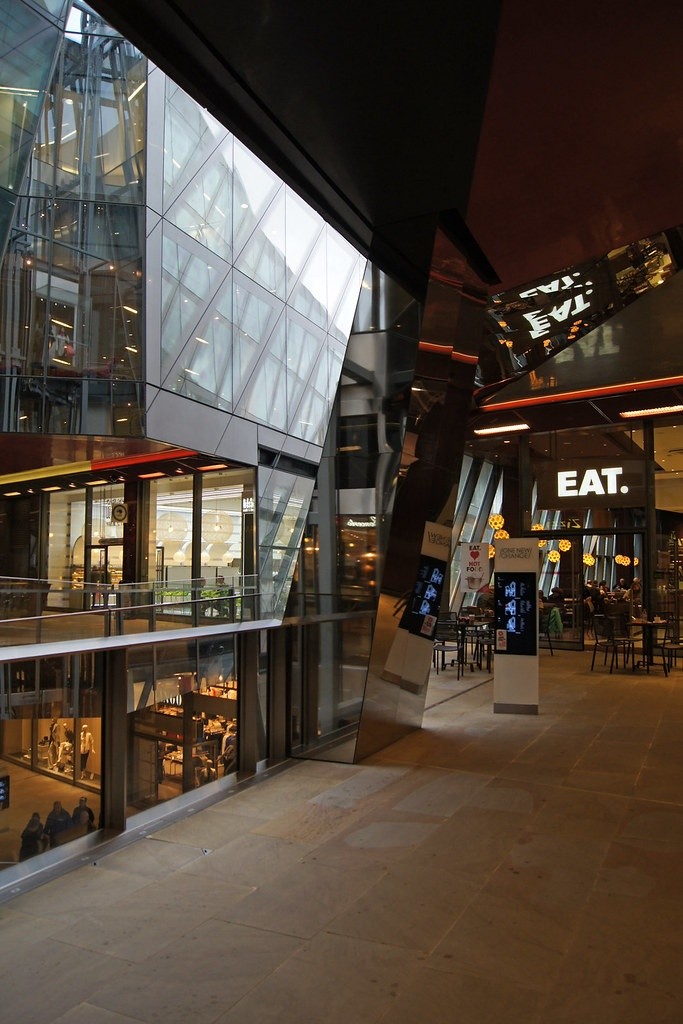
[459,622,489,666]
[625,621,671,673]
[163,756,183,777]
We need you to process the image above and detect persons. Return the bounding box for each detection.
[18,817,46,862]
[476,577,643,638]
[212,575,230,617]
[99,564,112,610]
[197,759,219,786]
[44,313,74,361]
[43,801,72,849]
[72,797,95,826]
[72,811,96,842]
[199,577,211,616]
[31,813,45,855]
[90,564,100,610]
[47,718,96,782]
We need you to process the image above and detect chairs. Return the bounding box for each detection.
[538,603,557,656]
[431,606,494,681]
[591,612,683,677]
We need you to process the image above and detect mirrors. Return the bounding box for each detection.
[354,342,479,765]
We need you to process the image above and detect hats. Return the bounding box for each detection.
[79,797,87,802]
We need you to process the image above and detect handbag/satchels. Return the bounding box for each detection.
[64,344,75,357]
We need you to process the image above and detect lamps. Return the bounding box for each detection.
[474,410,532,435]
[488,479,639,567]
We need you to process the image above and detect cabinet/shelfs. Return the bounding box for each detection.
[72,574,123,584]
